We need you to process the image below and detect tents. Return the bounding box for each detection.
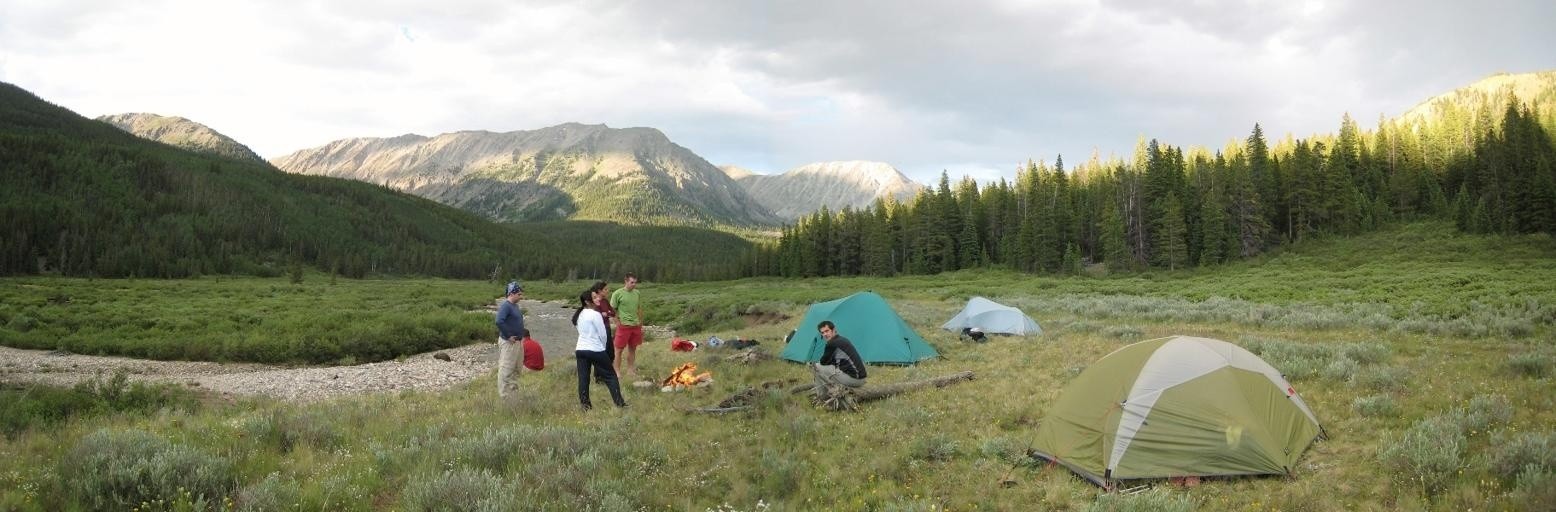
[1025,331,1321,492]
[780,291,943,365]
[942,295,1044,337]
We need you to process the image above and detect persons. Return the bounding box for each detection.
[522,328,546,372]
[590,281,617,382]
[573,291,627,409]
[609,273,643,380]
[495,281,525,398]
[811,321,868,400]
